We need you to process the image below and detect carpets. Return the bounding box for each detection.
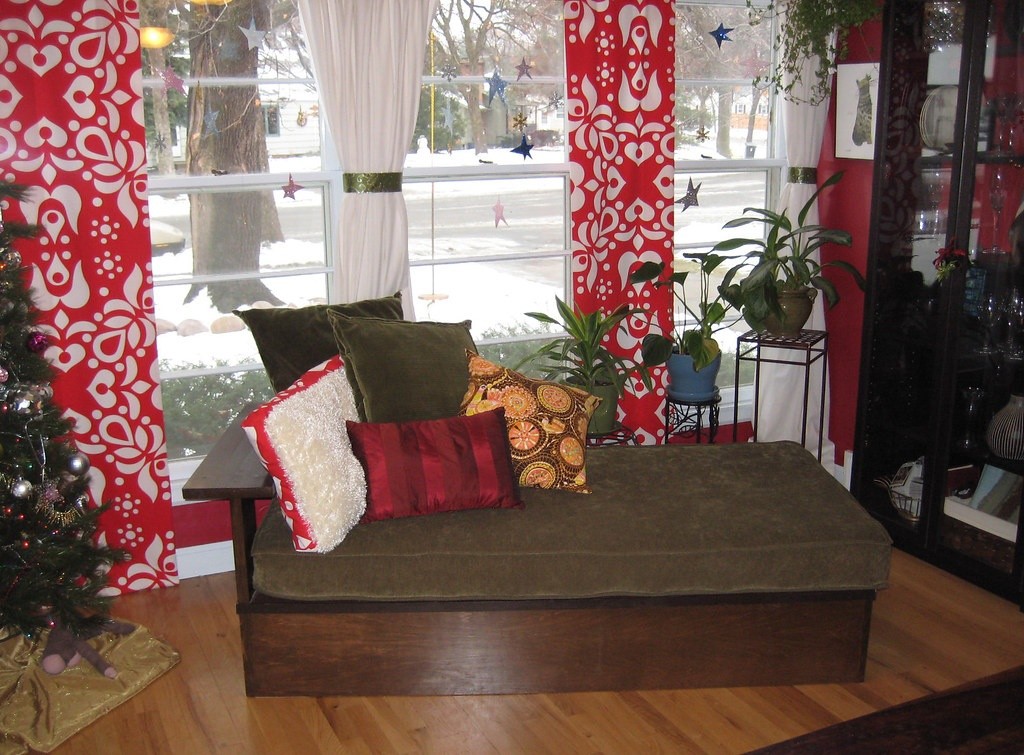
[742,662,1024,755]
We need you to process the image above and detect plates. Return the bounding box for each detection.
[919,84,959,150]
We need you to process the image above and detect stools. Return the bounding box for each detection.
[587,425,640,448]
[663,395,722,445]
[733,327,827,463]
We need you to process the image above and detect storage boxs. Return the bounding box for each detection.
[944,495,1021,542]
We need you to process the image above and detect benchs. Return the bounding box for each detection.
[183,400,890,694]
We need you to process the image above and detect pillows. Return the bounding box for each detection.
[242,355,367,554]
[324,309,479,423]
[456,348,604,494]
[346,405,524,526]
[232,288,404,394]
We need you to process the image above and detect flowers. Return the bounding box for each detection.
[934,237,968,285]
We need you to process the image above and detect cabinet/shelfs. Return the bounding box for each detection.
[850,0,1024,614]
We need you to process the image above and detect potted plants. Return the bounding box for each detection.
[631,251,765,403]
[511,294,655,434]
[709,170,867,338]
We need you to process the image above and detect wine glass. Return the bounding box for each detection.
[973,286,1024,361]
